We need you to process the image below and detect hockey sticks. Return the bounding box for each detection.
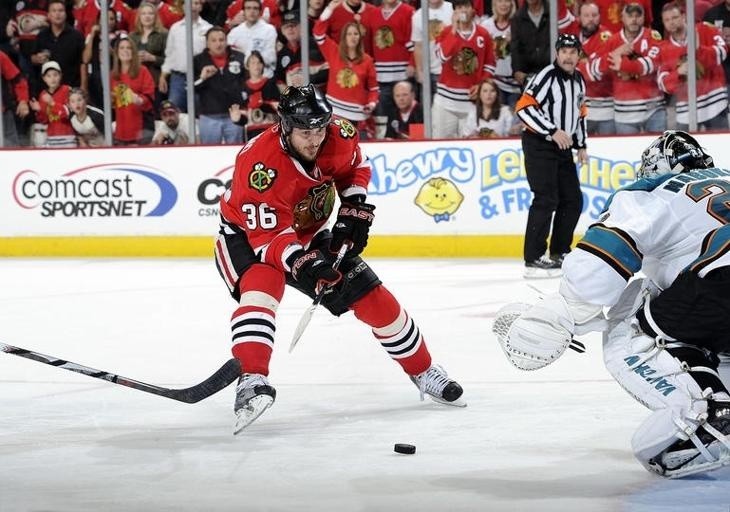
[2,343,242,405]
[289,241,348,350]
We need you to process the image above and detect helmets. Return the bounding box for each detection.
[636,128,714,175]
[554,33,581,50]
[277,82,334,130]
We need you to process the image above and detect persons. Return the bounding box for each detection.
[654,4,728,132]
[159,1,216,115]
[275,10,331,101]
[130,2,183,31]
[191,26,254,145]
[6,2,53,79]
[328,0,381,63]
[305,1,325,62]
[479,1,522,109]
[411,1,457,105]
[212,84,466,434]
[223,0,282,37]
[588,1,654,37]
[493,131,728,479]
[80,8,128,134]
[72,1,133,38]
[514,34,591,272]
[67,88,105,145]
[29,1,86,124]
[1,42,29,149]
[227,1,279,98]
[512,1,552,99]
[555,2,616,136]
[107,38,157,142]
[432,0,497,137]
[152,100,200,145]
[361,2,419,137]
[228,49,280,142]
[579,4,670,132]
[385,82,424,139]
[462,79,514,139]
[31,61,75,148]
[125,3,172,119]
[312,1,380,142]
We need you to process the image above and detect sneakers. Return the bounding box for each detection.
[411,368,464,401]
[660,399,730,460]
[232,373,277,414]
[550,252,565,263]
[524,256,562,270]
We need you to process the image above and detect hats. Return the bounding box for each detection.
[280,8,300,25]
[42,59,62,73]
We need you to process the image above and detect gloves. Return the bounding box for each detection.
[329,196,376,257]
[284,248,342,294]
